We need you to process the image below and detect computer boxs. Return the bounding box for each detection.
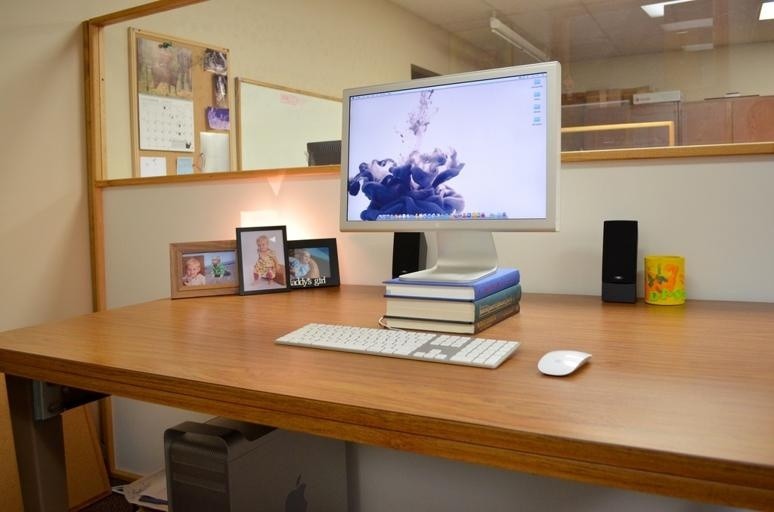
[164,415,348,512]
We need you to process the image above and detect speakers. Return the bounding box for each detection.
[392,232,427,278]
[602,220,638,304]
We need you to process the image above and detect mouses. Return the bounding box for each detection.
[537,349,592,376]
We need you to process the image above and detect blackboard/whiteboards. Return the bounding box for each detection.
[235,77,344,172]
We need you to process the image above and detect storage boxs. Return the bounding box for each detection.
[562,85,685,108]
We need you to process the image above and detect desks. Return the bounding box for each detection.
[0,287,774,512]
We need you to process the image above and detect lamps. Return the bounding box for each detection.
[490,10,553,63]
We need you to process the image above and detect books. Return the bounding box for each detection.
[381,266,522,333]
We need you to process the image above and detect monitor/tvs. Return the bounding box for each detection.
[340,60,560,283]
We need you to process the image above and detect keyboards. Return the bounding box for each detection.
[273,322,521,369]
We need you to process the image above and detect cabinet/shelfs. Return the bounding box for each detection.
[561,95,774,151]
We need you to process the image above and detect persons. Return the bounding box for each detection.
[289,251,311,280]
[253,236,279,285]
[177,46,192,91]
[210,256,231,280]
[181,258,205,286]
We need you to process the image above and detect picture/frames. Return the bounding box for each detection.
[287,239,341,290]
[170,240,239,298]
[236,225,291,295]
